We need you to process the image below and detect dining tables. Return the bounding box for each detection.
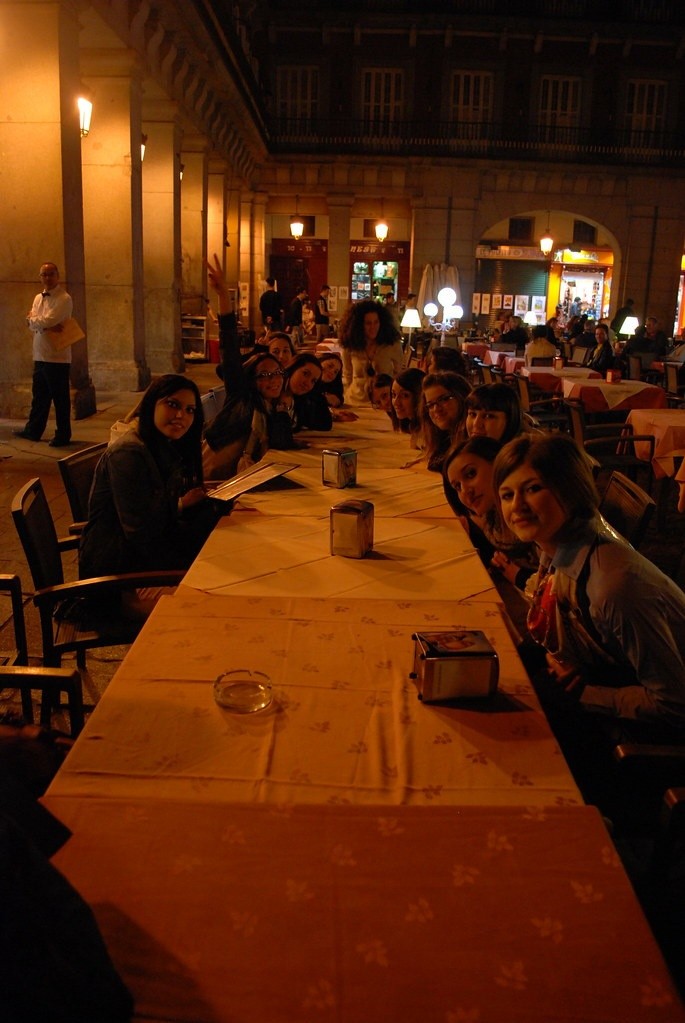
[521,366,601,433]
[463,342,525,386]
[562,377,666,462]
[617,409,685,510]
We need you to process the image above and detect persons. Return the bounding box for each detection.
[292,287,307,336]
[80,375,223,618]
[587,324,613,379]
[496,310,529,350]
[403,294,419,314]
[315,285,334,341]
[492,434,685,722]
[622,316,667,361]
[446,434,543,595]
[385,292,395,309]
[571,297,581,317]
[200,253,345,481]
[419,372,473,473]
[610,298,635,340]
[11,262,73,447]
[465,382,521,443]
[390,367,426,434]
[260,277,284,332]
[547,314,598,348]
[364,373,395,414]
[429,347,467,376]
[525,324,556,368]
[337,299,404,407]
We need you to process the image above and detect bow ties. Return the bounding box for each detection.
[42,292,51,297]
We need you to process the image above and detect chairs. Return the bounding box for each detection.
[24,383,685,1022]
[0,443,188,788]
[459,352,656,558]
[663,362,685,407]
[627,356,643,382]
[562,340,588,365]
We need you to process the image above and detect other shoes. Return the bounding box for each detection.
[12,428,39,441]
[49,437,69,446]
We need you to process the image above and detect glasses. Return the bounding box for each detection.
[424,394,454,413]
[40,271,56,276]
[251,370,284,380]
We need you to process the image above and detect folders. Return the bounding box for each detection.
[206,460,302,501]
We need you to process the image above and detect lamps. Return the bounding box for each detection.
[374,197,389,242]
[76,79,93,140]
[540,210,553,256]
[423,287,463,348]
[289,196,305,241]
[140,132,147,164]
[399,309,421,355]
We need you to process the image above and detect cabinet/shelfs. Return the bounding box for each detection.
[182,315,207,363]
